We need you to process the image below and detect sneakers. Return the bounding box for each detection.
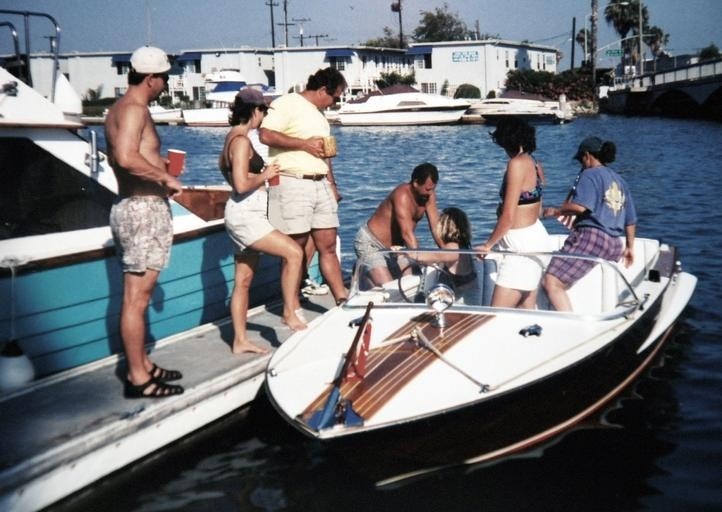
[300,274,330,298]
[295,308,307,324]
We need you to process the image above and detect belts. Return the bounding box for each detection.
[303,174,324,181]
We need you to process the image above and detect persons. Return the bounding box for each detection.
[395,206,481,306]
[470,120,549,310]
[350,163,445,287]
[218,89,306,356]
[540,135,639,312]
[257,68,349,326]
[103,43,185,399]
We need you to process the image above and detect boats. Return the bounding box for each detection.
[481,106,575,124]
[81,101,183,125]
[183,108,340,128]
[264,234,698,492]
[469,98,546,113]
[338,92,472,126]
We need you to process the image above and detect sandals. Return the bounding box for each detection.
[148,363,181,382]
[124,376,184,398]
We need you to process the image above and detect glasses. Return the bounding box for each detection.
[331,94,340,102]
[493,136,496,143]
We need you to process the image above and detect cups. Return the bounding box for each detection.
[166,149,186,177]
[321,136,336,158]
[265,165,279,187]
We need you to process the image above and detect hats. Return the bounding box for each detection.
[572,136,603,160]
[235,86,276,110]
[130,43,185,75]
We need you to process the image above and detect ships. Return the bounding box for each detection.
[0,7,340,378]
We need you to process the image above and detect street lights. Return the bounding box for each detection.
[584,2,628,63]
